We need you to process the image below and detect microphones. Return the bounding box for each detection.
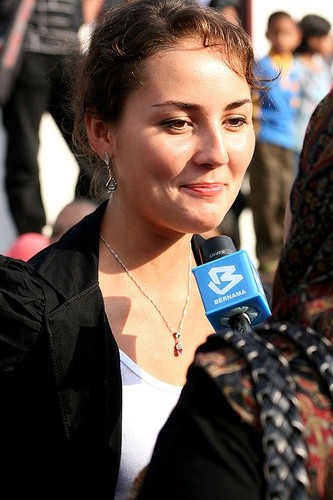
[200,233,252,338]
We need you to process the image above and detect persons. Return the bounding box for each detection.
[0,0,281,500]
[196,0,332,285]
[129,87,333,500]
[0,0,133,262]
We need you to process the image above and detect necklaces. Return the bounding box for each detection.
[98,232,194,354]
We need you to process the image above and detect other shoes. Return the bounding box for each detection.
[257,267,279,284]
